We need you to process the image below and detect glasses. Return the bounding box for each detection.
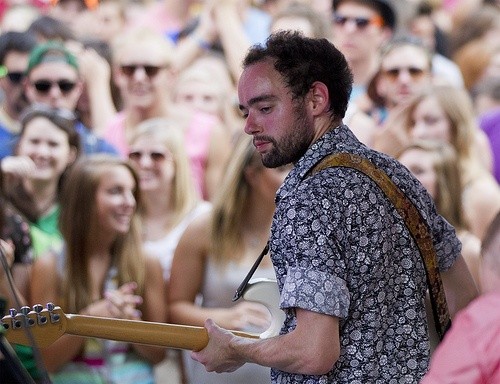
[29,78,79,94]
[128,152,165,164]
[381,66,424,79]
[335,15,382,30]
[121,64,159,77]
[5,72,24,82]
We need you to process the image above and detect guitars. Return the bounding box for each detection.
[0,303,263,352]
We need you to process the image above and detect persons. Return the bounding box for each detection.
[32,152,167,384]
[125,116,214,384]
[168,130,295,384]
[0,0,327,314]
[191,30,480,384]
[328,0,500,295]
